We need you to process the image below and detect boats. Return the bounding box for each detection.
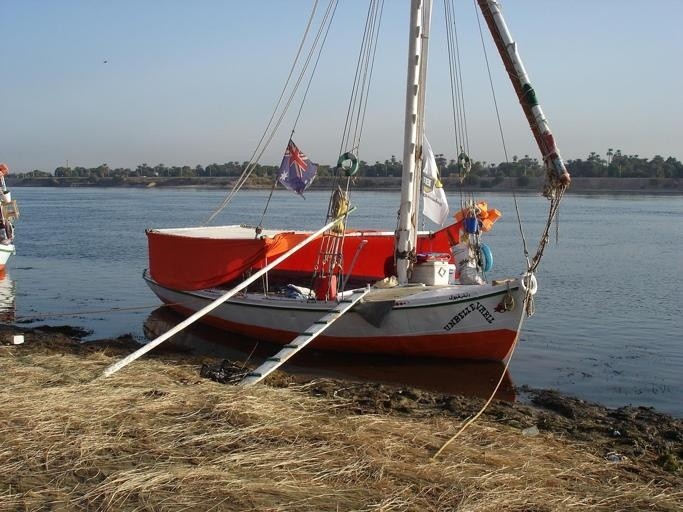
[141,307,518,416]
[0,264,16,319]
[0,162,20,270]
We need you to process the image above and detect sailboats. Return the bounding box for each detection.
[140,3,572,362]
